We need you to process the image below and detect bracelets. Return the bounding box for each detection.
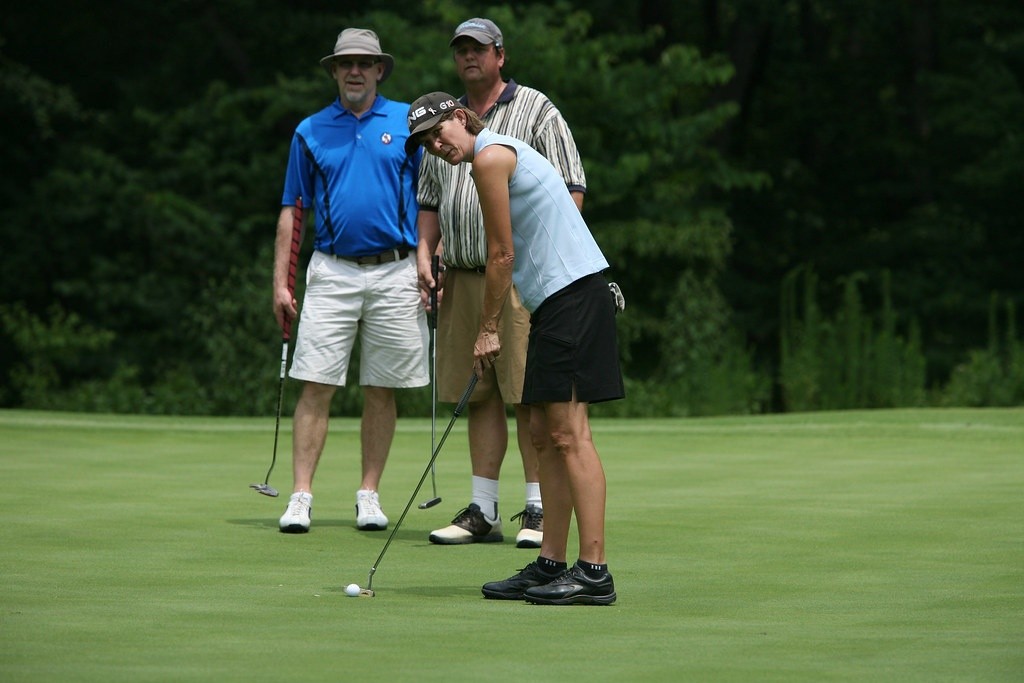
[437,265,447,273]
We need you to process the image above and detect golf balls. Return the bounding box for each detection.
[347,583,360,596]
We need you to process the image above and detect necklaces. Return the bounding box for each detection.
[473,134,476,142]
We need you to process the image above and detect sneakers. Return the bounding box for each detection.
[523,562,617,605]
[429,503,503,544]
[481,562,568,600]
[510,504,543,548]
[355,490,389,530]
[279,489,313,533]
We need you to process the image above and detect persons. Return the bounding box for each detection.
[273,26,446,532]
[404,92,627,606]
[414,17,587,547]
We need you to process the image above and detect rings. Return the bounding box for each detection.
[490,359,495,362]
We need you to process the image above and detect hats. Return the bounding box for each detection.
[320,28,393,82]
[404,92,466,156]
[449,18,503,47]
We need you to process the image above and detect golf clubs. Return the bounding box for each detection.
[365,366,488,599]
[419,255,442,511]
[250,196,305,497]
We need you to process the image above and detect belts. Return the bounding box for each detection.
[338,243,412,266]
